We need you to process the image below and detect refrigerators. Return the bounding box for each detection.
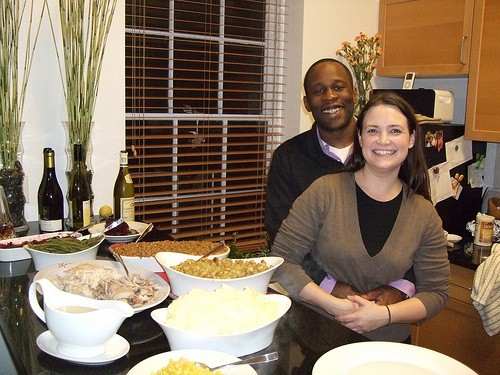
[413,123,489,246]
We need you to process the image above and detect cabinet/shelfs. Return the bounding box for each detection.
[411,269,500,375]
[374,0,500,143]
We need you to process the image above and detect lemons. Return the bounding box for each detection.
[99,205,112,216]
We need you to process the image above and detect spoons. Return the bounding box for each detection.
[192,352,279,372]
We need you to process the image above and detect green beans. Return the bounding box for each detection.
[28,234,103,254]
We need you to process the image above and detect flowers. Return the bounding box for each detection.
[331,28,386,82]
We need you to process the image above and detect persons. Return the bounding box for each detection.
[267,58,434,305]
[437,131,443,151]
[268,93,451,375]
[426,134,437,147]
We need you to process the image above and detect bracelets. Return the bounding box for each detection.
[386,305,391,326]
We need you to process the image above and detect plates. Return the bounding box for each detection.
[0,232,82,262]
[33,260,171,314]
[87,219,153,243]
[124,351,259,375]
[311,340,478,375]
[447,233,463,242]
[36,330,131,363]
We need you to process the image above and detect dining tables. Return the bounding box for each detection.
[0,215,371,375]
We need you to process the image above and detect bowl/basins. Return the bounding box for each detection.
[22,233,106,271]
[154,252,283,296]
[149,294,291,356]
[108,243,231,274]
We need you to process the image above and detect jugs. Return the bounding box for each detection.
[29,278,134,358]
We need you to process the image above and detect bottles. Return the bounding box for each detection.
[37,148,64,232]
[68,144,91,231]
[0,185,16,241]
[113,150,135,222]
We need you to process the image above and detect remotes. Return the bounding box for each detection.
[403,72,416,90]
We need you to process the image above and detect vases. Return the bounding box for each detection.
[353,80,374,116]
[0,121,28,231]
[62,121,95,227]
